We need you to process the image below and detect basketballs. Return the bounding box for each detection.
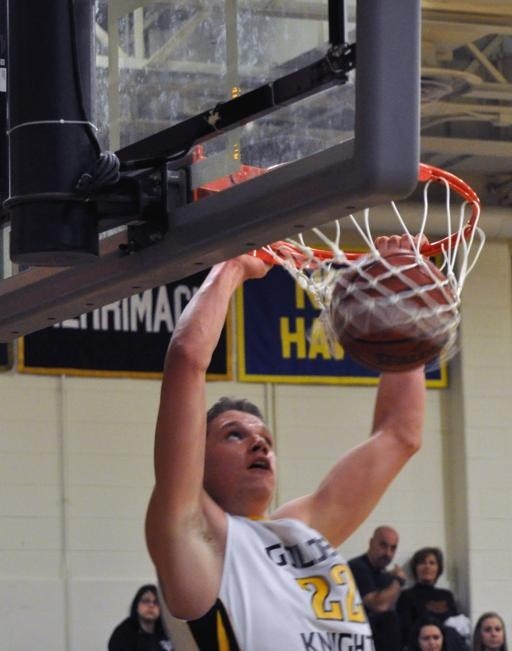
[330,248,455,375]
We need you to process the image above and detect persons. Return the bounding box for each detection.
[108,584,173,651]
[406,614,449,651]
[348,526,406,651]
[144,234,430,651]
[473,612,507,651]
[397,547,457,646]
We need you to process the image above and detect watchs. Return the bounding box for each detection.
[393,575,405,585]
